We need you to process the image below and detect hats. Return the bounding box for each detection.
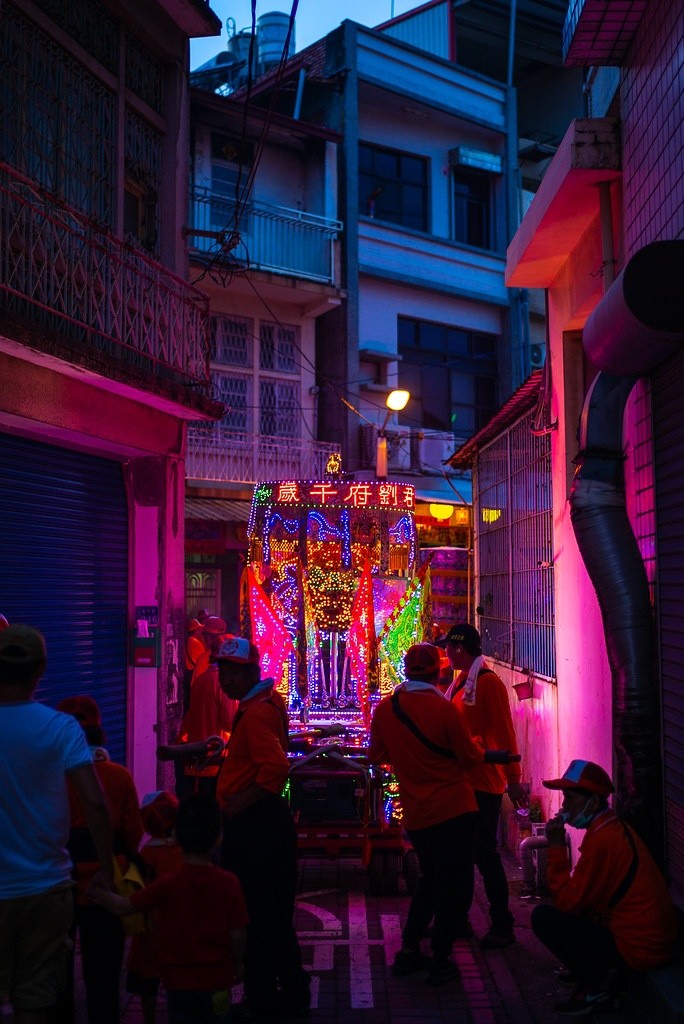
[404,645,437,675]
[437,624,481,647]
[0,624,47,661]
[215,638,259,665]
[57,696,101,726]
[542,759,612,797]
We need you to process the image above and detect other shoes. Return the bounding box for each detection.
[558,970,580,986]
[424,925,475,938]
[480,929,518,947]
[555,985,620,1015]
[393,950,433,973]
[429,959,459,985]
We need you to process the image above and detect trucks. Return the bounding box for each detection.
[289,757,420,896]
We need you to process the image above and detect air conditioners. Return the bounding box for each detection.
[531,342,547,368]
[360,423,411,471]
[410,429,454,477]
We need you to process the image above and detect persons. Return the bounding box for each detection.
[529,758,679,1016]
[367,644,480,984]
[208,638,313,1024]
[423,622,528,949]
[55,695,182,1024]
[155,609,240,798]
[0,624,115,1024]
[84,794,250,1024]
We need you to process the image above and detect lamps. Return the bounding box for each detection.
[380,389,410,436]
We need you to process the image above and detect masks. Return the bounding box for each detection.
[566,797,595,829]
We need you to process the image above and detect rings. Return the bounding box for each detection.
[520,795,524,800]
